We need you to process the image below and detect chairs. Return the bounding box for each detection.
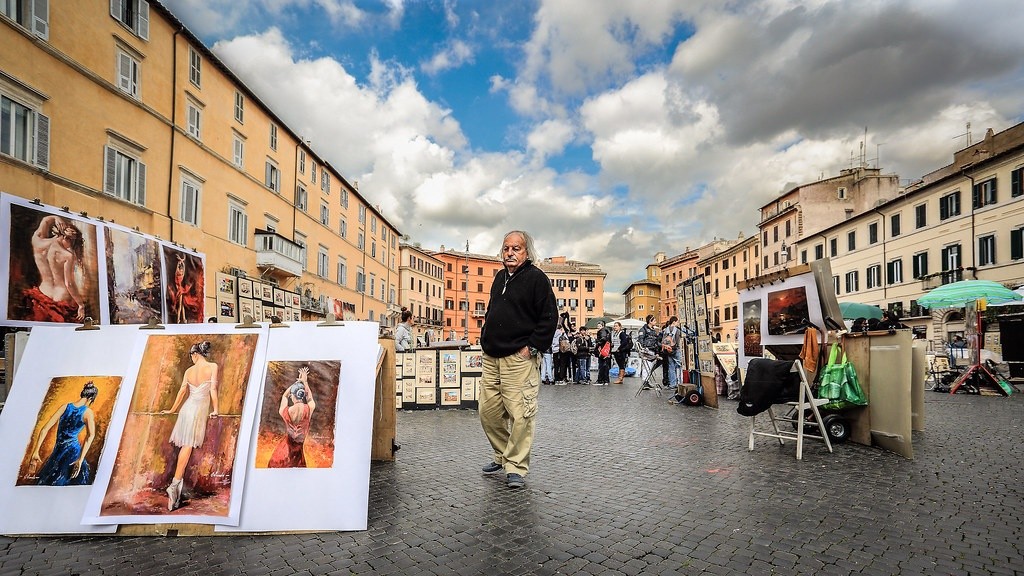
[924,361,950,392]
[737,359,833,460]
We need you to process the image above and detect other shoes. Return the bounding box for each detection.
[507,473,525,487]
[592,381,603,385]
[604,382,608,386]
[481,462,503,474]
[541,376,592,385]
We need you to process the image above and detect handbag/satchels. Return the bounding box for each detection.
[818,343,869,409]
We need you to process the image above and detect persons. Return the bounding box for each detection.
[538,311,684,392]
[850,311,909,333]
[395,307,414,352]
[949,335,967,348]
[475,230,559,490]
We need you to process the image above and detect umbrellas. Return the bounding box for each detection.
[838,300,883,323]
[916,278,1024,366]
[603,317,660,331]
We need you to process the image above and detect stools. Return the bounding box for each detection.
[633,341,665,398]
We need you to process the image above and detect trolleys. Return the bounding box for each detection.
[783,313,851,443]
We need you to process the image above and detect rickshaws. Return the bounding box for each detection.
[667,325,703,406]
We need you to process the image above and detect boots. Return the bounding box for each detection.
[614,369,625,384]
